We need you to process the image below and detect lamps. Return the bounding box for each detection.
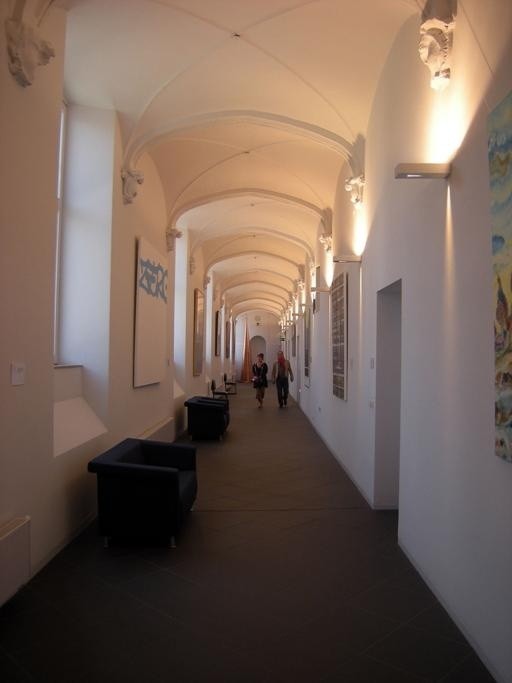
[333,255,362,263]
[300,304,313,307]
[278,313,304,333]
[310,287,330,292]
[394,163,451,179]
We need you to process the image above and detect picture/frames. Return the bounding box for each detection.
[134,238,169,389]
[216,311,220,356]
[226,321,230,358]
[193,288,204,375]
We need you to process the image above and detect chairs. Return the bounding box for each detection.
[88,438,197,548]
[211,379,228,400]
[185,396,229,443]
[224,373,236,394]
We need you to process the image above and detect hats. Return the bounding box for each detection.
[256,353,264,360]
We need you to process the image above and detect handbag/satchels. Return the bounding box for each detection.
[251,376,259,388]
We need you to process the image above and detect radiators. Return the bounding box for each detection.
[0,518,31,606]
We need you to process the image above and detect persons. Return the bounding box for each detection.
[271,349,295,408]
[250,351,269,408]
